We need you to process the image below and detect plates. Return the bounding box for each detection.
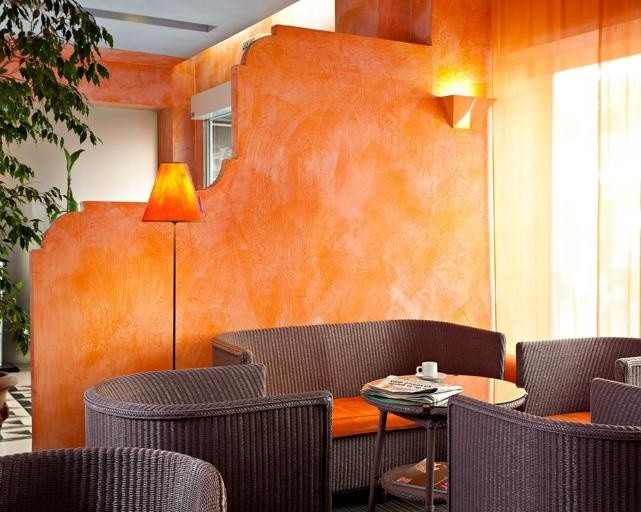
[415,372,446,380]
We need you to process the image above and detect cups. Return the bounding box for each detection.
[416,362,438,378]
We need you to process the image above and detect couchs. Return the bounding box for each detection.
[211,319,505,492]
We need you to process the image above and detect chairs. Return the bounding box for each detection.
[84,365,333,511]
[0,447,226,512]
[448,377,640,512]
[516,338,641,424]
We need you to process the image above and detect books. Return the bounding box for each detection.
[392,456,449,494]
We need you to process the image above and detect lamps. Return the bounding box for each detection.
[436,93,496,129]
[141,162,206,369]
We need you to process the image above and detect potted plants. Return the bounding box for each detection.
[0,268,32,422]
